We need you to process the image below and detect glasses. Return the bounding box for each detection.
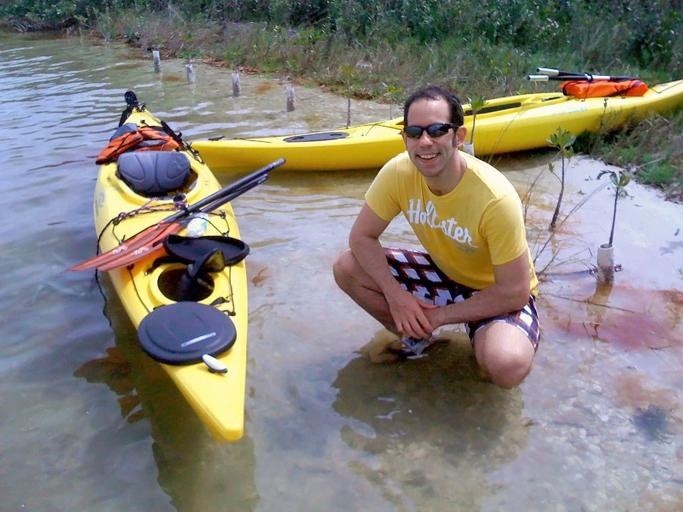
[404,123,458,138]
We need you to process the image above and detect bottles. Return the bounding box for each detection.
[188,210,208,237]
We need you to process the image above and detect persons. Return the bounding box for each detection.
[330,85,541,391]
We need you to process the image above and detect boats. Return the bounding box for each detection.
[185,77,682,169]
[93,105,251,451]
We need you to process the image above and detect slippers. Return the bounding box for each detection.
[369,339,450,366]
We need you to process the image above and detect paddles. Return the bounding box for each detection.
[66,158,286,271]
